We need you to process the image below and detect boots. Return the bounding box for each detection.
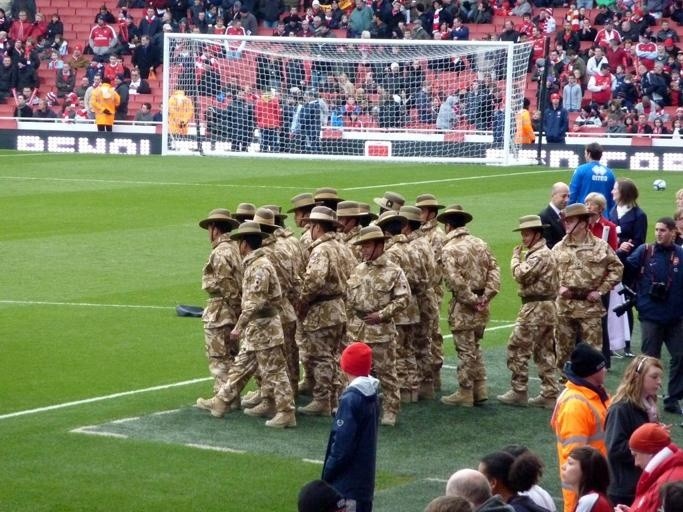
[473,381,487,402]
[243,399,275,418]
[497,390,527,407]
[297,378,311,395]
[440,385,473,407]
[379,393,411,404]
[528,394,555,410]
[240,391,262,407]
[419,384,434,399]
[197,397,228,417]
[381,411,396,426]
[265,410,296,428]
[297,399,331,416]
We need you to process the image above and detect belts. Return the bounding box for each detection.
[209,290,223,298]
[566,286,592,300]
[310,294,340,303]
[409,288,421,295]
[452,289,484,299]
[249,306,277,320]
[522,296,551,305]
[355,309,367,320]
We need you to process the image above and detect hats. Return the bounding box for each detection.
[617,92,625,98]
[373,191,405,214]
[230,203,256,216]
[163,23,172,31]
[677,50,683,54]
[229,220,270,241]
[312,0,320,6]
[437,204,473,223]
[604,18,612,23]
[352,225,391,246]
[412,20,422,24]
[97,14,105,20]
[314,188,345,200]
[119,10,127,15]
[558,203,597,221]
[91,55,100,62]
[115,73,124,80]
[663,66,672,73]
[591,103,598,110]
[199,208,240,229]
[360,202,379,220]
[337,201,368,217]
[261,205,287,218]
[47,91,56,102]
[287,193,315,213]
[244,208,282,228]
[339,341,373,376]
[628,423,671,454]
[73,44,82,51]
[298,205,346,228]
[398,205,424,222]
[415,193,446,208]
[546,7,553,14]
[520,97,530,106]
[640,58,654,70]
[665,39,672,47]
[570,342,607,378]
[551,93,560,99]
[375,210,407,229]
[512,214,550,232]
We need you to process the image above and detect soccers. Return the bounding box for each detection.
[653,178,666,192]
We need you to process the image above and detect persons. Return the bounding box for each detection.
[675,189,683,209]
[374,192,405,216]
[196,220,297,428]
[608,177,648,256]
[270,1,501,94]
[567,142,615,220]
[615,217,682,416]
[623,116,636,133]
[674,210,683,248]
[651,118,670,134]
[400,206,444,400]
[605,355,673,508]
[669,119,683,134]
[314,187,345,211]
[539,181,573,248]
[572,103,602,131]
[478,451,552,512]
[0,1,94,124]
[297,206,359,420]
[635,114,652,133]
[606,115,626,138]
[578,18,617,104]
[231,203,258,223]
[551,203,624,389]
[635,96,656,121]
[614,422,682,511]
[586,192,631,354]
[225,90,535,153]
[436,205,501,407]
[85,1,285,133]
[374,210,428,403]
[493,1,585,150]
[200,207,241,410]
[672,108,683,120]
[445,468,514,512]
[657,481,683,512]
[321,342,381,512]
[288,192,324,393]
[648,101,669,122]
[562,446,612,512]
[590,104,603,120]
[337,202,365,263]
[551,342,612,511]
[264,205,299,246]
[594,0,683,104]
[344,226,410,428]
[496,213,558,410]
[602,100,625,124]
[503,445,556,510]
[415,194,446,304]
[242,207,298,419]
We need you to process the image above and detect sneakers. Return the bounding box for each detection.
[624,351,635,358]
[608,349,624,359]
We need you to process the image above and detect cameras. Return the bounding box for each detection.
[612,284,638,317]
